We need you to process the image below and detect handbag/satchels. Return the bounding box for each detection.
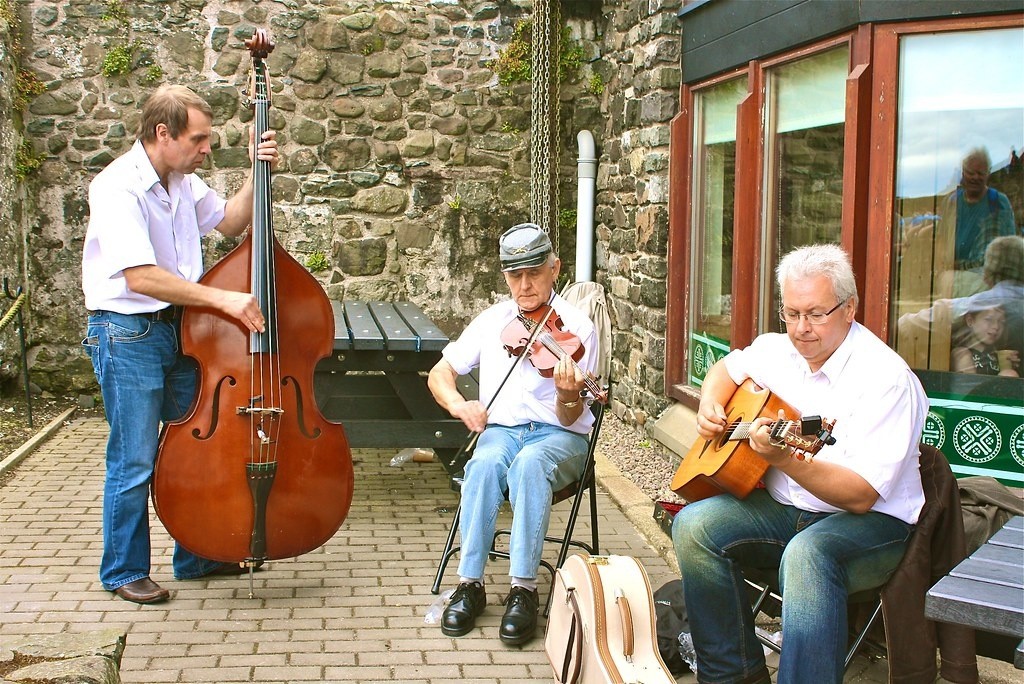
[653,578,697,675]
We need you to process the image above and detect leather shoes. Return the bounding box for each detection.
[116,577,169,604]
[206,562,262,576]
[499,586,539,644]
[441,581,487,637]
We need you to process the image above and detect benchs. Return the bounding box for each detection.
[454,366,480,402]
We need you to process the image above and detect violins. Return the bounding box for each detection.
[500,301,612,407]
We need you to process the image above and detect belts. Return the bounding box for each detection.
[87,306,180,322]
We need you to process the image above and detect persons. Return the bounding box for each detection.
[82,85,281,602]
[428,224,598,646]
[672,246,930,684]
[952,299,1024,379]
[899,147,1024,351]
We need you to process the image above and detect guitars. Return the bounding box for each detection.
[671,377,838,503]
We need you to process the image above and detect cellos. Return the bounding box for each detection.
[149,26,355,601]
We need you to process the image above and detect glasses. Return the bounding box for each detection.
[779,296,850,325]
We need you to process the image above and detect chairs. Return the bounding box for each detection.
[432,380,605,618]
[744,445,951,678]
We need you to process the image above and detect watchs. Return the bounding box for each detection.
[557,393,580,408]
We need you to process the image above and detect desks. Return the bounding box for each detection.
[923,514,1024,683]
[312,299,478,491]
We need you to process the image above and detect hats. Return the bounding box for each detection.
[499,223,551,273]
